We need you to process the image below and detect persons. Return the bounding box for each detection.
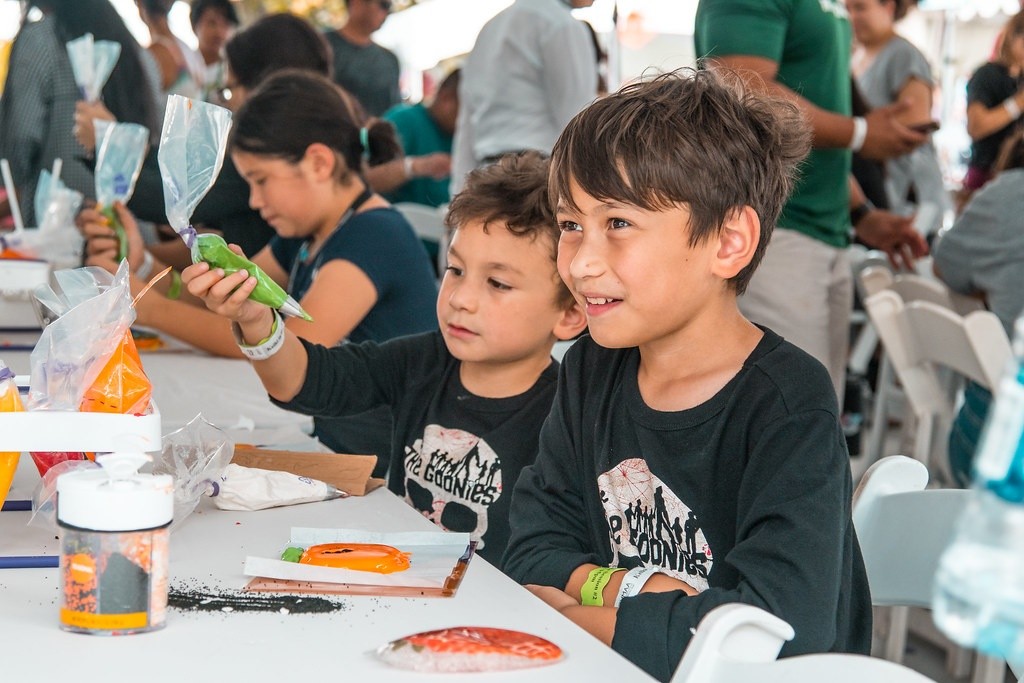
[81,68,440,479]
[1,0,441,358]
[498,40,852,683]
[181,147,588,568]
[693,0,1024,506]
[357,0,610,206]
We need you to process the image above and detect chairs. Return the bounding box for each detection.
[395,202,1024,683]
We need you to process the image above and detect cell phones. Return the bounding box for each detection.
[907,119,940,134]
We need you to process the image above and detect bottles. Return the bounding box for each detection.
[932,352,1024,659]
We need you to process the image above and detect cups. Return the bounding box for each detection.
[0,258,50,330]
[56,471,169,635]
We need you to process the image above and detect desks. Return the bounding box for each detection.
[0,309,650,683]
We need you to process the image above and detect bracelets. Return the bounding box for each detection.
[581,567,630,607]
[846,115,868,153]
[851,201,876,228]
[613,566,669,607]
[1004,96,1020,121]
[163,269,181,299]
[134,251,154,280]
[230,308,285,360]
[404,157,415,178]
